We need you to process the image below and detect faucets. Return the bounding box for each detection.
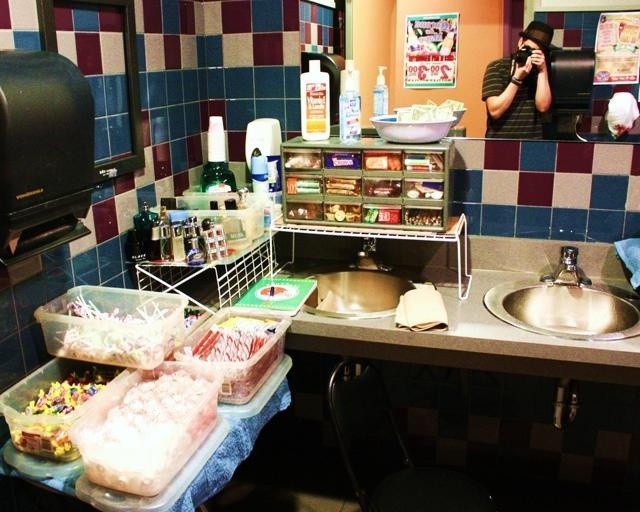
[541,247,592,288]
[350,237,393,272]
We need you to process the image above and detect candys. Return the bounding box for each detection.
[13,294,279,496]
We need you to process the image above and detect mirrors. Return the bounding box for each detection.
[345,0,640,145]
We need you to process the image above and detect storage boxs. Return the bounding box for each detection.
[186,306,215,336]
[279,135,455,234]
[2,354,139,464]
[174,307,291,405]
[66,362,223,497]
[32,286,190,370]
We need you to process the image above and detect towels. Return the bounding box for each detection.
[614,238,640,293]
[395,287,450,333]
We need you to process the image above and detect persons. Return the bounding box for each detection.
[480,20,554,140]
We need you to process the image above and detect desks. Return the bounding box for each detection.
[0,353,297,511]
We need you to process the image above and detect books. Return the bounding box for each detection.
[232,276,319,316]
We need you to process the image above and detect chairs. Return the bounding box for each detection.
[326,355,500,512]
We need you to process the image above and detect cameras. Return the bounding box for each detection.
[515,45,536,66]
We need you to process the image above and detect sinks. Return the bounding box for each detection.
[483,280,640,341]
[294,267,437,321]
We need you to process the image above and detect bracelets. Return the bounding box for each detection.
[510,76,523,86]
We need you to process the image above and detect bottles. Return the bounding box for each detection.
[132,200,228,263]
[199,115,238,211]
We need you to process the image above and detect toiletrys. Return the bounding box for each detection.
[120,202,227,265]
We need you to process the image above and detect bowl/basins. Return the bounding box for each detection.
[392,107,469,129]
[368,113,459,145]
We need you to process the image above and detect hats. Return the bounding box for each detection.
[518,20,563,49]
